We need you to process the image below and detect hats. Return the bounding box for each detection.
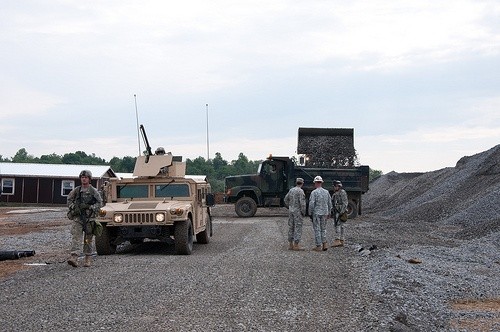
[297,178,304,183]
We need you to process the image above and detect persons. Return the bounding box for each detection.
[67,170,101,267]
[308,176,332,251]
[155,147,165,155]
[331,180,348,247]
[284,178,306,251]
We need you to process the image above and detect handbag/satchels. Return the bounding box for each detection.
[340,211,347,222]
[93,221,103,237]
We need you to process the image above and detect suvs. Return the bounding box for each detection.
[95,125,216,255]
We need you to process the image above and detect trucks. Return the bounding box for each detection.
[223,154,370,218]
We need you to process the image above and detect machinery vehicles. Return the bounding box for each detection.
[297,127,354,158]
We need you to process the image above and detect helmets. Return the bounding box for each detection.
[313,176,324,183]
[332,180,342,187]
[79,170,92,179]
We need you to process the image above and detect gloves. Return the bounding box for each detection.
[80,202,89,209]
[74,207,81,216]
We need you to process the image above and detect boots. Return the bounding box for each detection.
[332,239,341,246]
[293,241,305,251]
[84,255,91,266]
[323,242,332,250]
[67,255,78,267]
[340,240,345,246]
[312,245,322,251]
[288,242,293,250]
